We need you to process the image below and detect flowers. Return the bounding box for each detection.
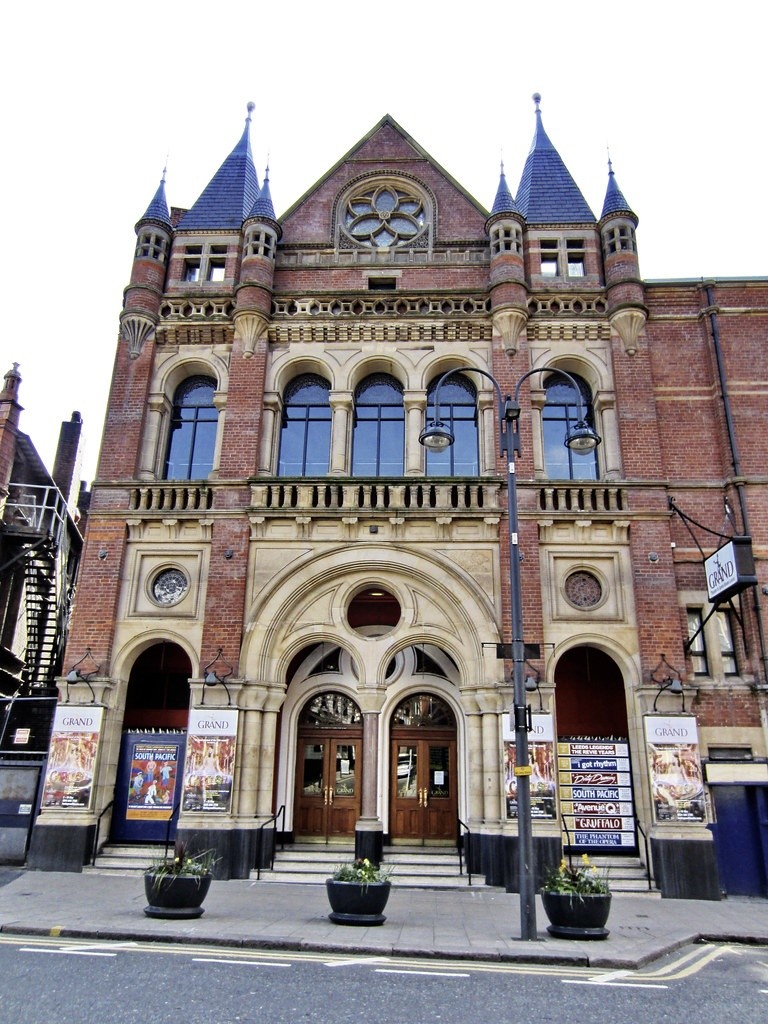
[142,839,223,898]
[333,857,396,897]
[543,854,612,912]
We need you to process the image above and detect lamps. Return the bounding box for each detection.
[650,653,687,713]
[65,647,101,703]
[199,648,233,706]
[510,659,543,711]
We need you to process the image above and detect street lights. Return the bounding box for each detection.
[417,367,602,942]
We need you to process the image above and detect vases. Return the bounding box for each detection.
[143,872,213,920]
[326,878,391,927]
[539,887,612,940]
